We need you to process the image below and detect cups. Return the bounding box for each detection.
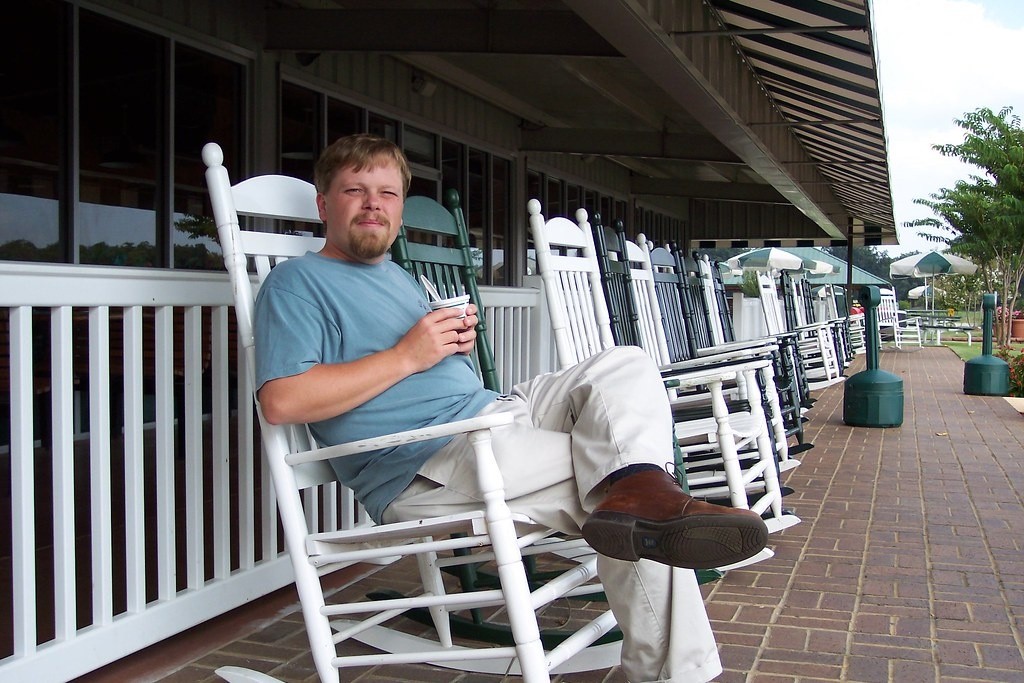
[429,294,471,354]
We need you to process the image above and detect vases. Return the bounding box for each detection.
[992,323,1009,338]
[1011,319,1024,338]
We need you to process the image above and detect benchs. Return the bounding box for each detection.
[923,326,975,347]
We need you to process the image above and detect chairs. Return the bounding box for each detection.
[194,140,924,683]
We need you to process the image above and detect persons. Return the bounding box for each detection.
[255,133,768,683]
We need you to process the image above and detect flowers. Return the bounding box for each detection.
[992,306,1011,322]
[1012,310,1024,320]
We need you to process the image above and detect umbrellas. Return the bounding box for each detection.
[723,247,840,276]
[890,249,979,326]
[908,285,946,308]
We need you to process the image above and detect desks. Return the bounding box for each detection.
[937,319,960,335]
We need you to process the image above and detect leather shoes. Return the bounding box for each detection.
[582,462,769,571]
